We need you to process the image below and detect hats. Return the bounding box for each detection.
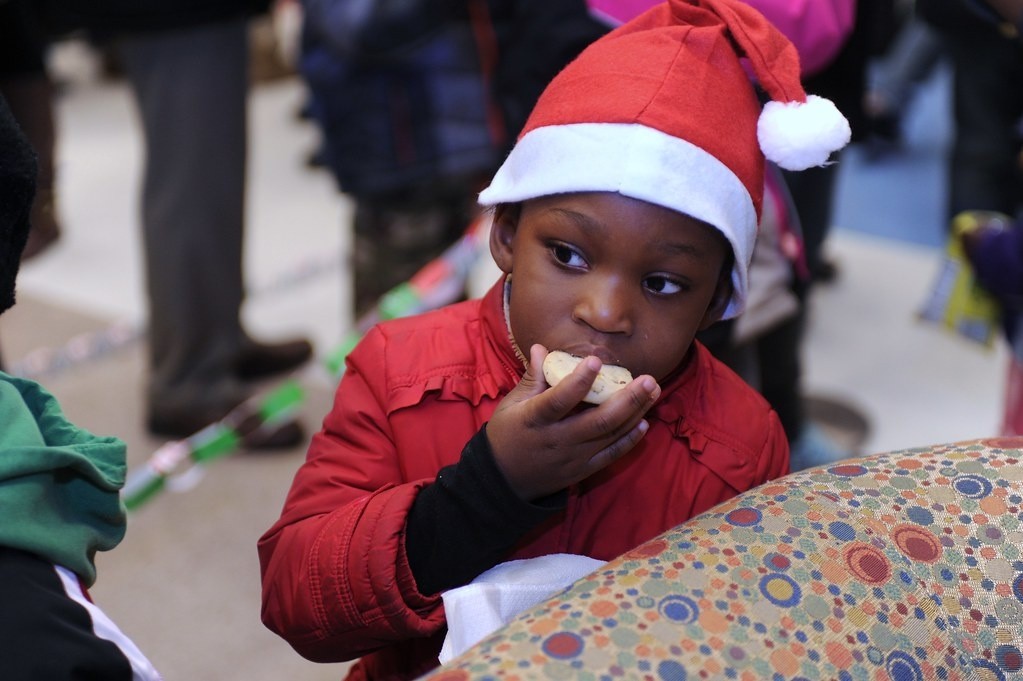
[478,0,852,320]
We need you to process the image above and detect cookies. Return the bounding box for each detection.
[543,350,633,405]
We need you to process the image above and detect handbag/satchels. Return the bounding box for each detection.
[319,48,432,198]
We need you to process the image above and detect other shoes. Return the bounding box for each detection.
[237,341,309,378]
[147,392,302,446]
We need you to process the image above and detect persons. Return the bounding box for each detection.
[0,0,314,449]
[258,0,852,681]
[294,1,1022,472]
[1,98,146,681]
